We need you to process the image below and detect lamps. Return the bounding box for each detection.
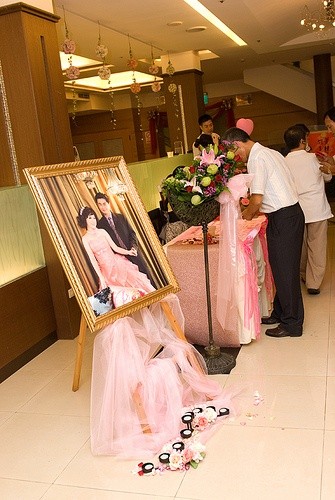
[298,0,335,35]
[104,167,127,201]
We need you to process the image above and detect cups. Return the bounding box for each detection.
[174,141,183,154]
[166,151,174,158]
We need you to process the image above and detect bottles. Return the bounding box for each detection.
[203,92,208,104]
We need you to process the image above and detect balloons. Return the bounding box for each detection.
[236,118,254,135]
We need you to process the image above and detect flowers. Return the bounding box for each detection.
[157,144,242,212]
[240,195,252,210]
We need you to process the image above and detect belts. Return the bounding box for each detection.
[265,206,288,218]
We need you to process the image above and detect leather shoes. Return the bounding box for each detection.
[261,315,281,324]
[265,324,302,337]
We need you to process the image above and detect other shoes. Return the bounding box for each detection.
[308,289,320,294]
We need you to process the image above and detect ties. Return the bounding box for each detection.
[107,217,127,250]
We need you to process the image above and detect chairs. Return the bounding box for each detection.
[160,221,187,246]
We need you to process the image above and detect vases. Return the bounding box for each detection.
[169,189,228,228]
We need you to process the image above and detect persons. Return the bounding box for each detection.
[277,128,334,295]
[292,123,314,153]
[75,192,156,310]
[192,114,220,159]
[315,107,335,175]
[194,134,215,157]
[219,127,305,337]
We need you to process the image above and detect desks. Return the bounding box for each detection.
[162,213,272,353]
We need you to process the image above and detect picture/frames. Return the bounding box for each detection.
[20,153,183,330]
